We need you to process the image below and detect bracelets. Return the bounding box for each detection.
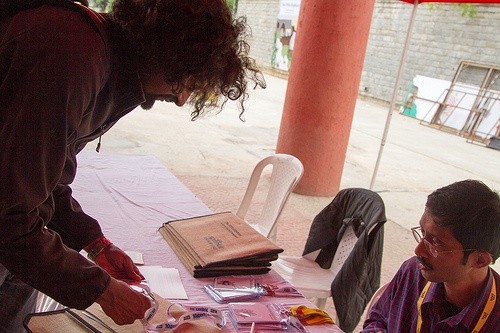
[87,237,112,261]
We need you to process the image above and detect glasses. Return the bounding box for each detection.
[412,226,480,257]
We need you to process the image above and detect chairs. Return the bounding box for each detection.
[269,188,387,333]
[236,153,305,239]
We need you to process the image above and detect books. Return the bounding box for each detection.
[126,210,284,332]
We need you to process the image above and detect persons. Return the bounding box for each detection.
[360,180,500,333]
[0,0,267,326]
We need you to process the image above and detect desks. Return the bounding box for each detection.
[1,150,347,333]
